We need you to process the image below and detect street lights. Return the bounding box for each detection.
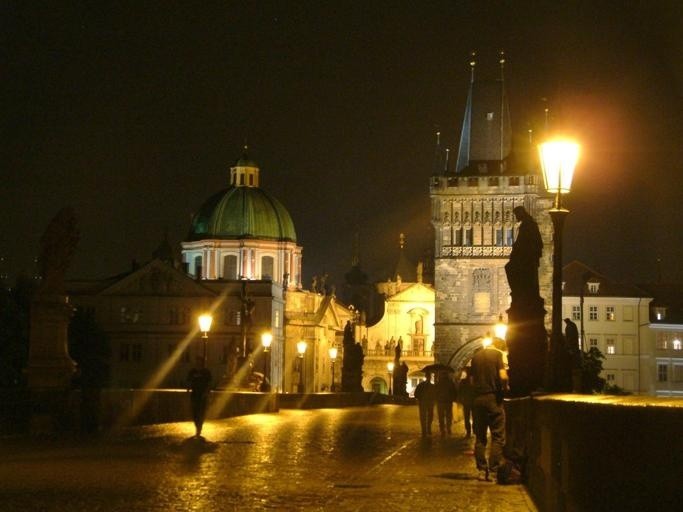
[260,330,273,388]
[534,125,581,387]
[294,340,307,393]
[198,314,213,369]
[495,321,509,354]
[384,361,394,395]
[325,348,337,392]
[484,329,492,348]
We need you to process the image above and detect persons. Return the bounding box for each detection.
[186,356,211,436]
[564,318,579,351]
[470,337,510,480]
[415,366,475,437]
[395,344,401,362]
[505,207,544,313]
[255,376,263,391]
[344,320,352,343]
[362,335,404,355]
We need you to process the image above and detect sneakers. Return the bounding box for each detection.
[478,471,499,482]
